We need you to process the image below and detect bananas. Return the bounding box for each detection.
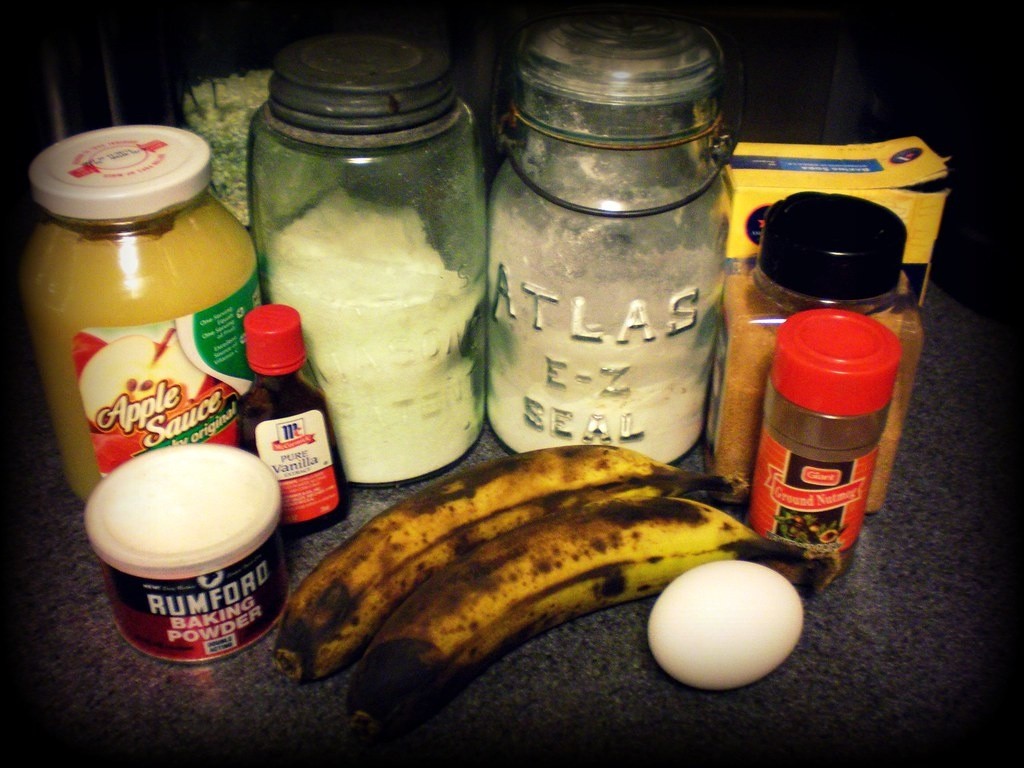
[347,492,838,737]
[276,443,749,680]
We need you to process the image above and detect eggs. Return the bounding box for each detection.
[648,559,804,690]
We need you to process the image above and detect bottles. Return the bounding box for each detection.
[238,304,352,540]
[705,191,923,515]
[21,124,260,498]
[248,29,490,487]
[751,307,903,573]
[82,441,291,665]
[486,15,732,465]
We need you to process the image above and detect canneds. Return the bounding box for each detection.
[18,125,262,506]
[83,443,285,666]
[712,192,926,558]
